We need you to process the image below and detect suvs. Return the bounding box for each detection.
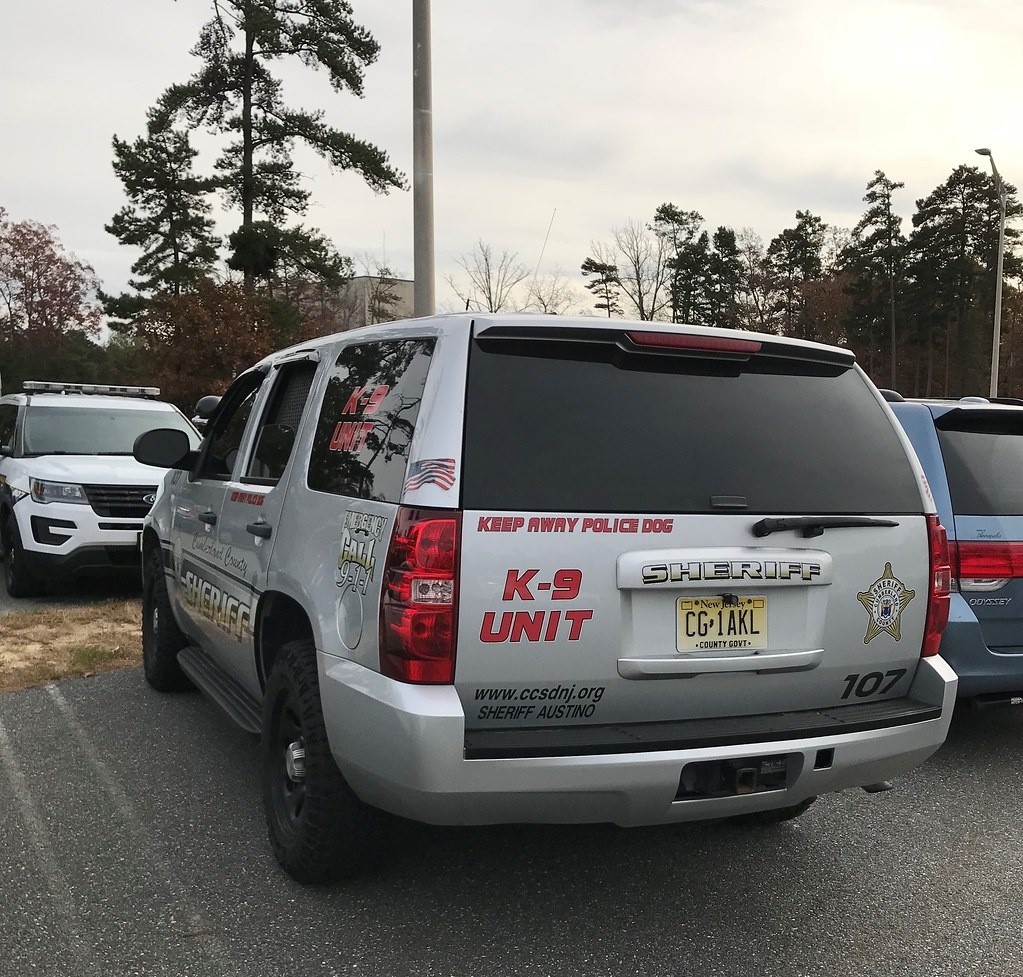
[876,392,1020,736]
[138,310,957,888]
[0,381,206,600]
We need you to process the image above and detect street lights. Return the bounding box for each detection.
[974,148,1008,400]
